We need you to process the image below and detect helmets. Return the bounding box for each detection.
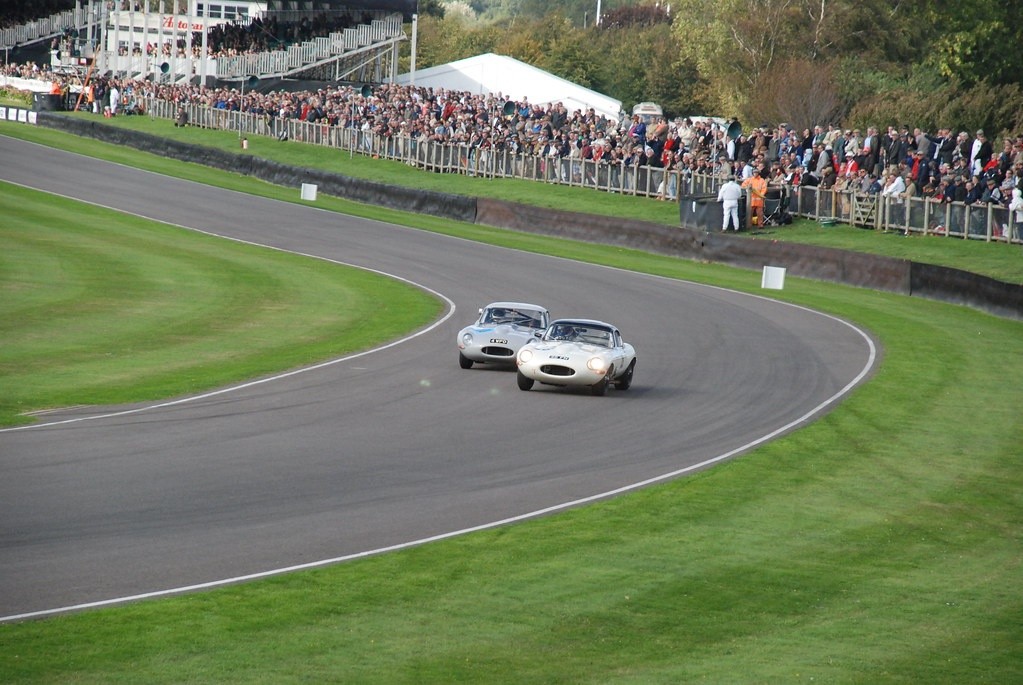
[491,307,506,319]
[557,325,574,338]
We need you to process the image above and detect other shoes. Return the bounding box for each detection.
[721,230,726,233]
[759,227,764,229]
[734,230,739,233]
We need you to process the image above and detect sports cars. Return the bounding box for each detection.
[457,301,553,368]
[516,320,636,394]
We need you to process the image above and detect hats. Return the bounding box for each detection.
[835,130,841,134]
[890,130,899,136]
[853,129,860,134]
[987,180,995,186]
[845,151,855,157]
[868,174,876,178]
[902,125,909,130]
[727,175,735,179]
[952,156,959,162]
[954,176,961,181]
[789,165,797,169]
[977,129,984,136]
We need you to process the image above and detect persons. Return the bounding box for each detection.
[491,309,506,321]
[93,81,105,114]
[716,175,742,234]
[0,0,1023,245]
[741,168,768,230]
[109,84,121,117]
[555,326,575,341]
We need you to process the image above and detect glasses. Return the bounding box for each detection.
[861,171,866,173]
[917,154,923,156]
